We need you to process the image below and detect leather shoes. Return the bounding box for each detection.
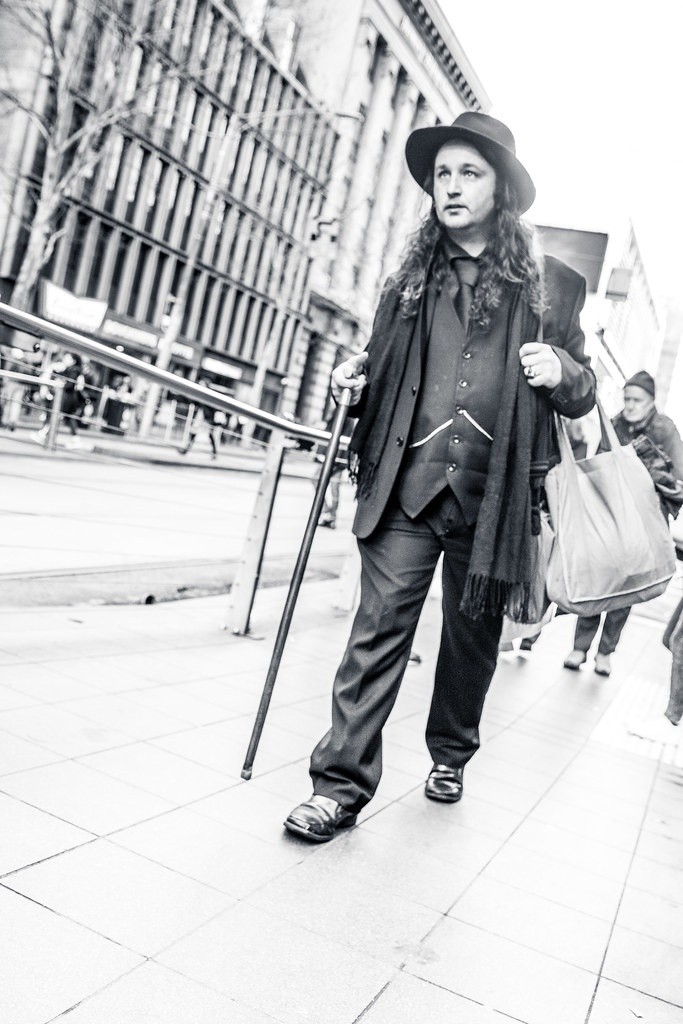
[425,762,464,801]
[283,793,358,841]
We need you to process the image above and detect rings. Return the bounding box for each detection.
[524,365,536,378]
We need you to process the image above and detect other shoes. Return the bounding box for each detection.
[519,639,532,656]
[565,649,587,669]
[593,654,611,674]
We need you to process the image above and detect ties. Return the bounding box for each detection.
[451,257,482,335]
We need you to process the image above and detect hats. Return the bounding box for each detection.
[405,111,535,216]
[623,371,656,397]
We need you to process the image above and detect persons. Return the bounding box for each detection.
[0,330,225,460]
[494,364,588,661]
[563,369,683,675]
[278,112,595,845]
[311,405,355,530]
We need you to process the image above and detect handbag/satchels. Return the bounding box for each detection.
[543,391,676,617]
